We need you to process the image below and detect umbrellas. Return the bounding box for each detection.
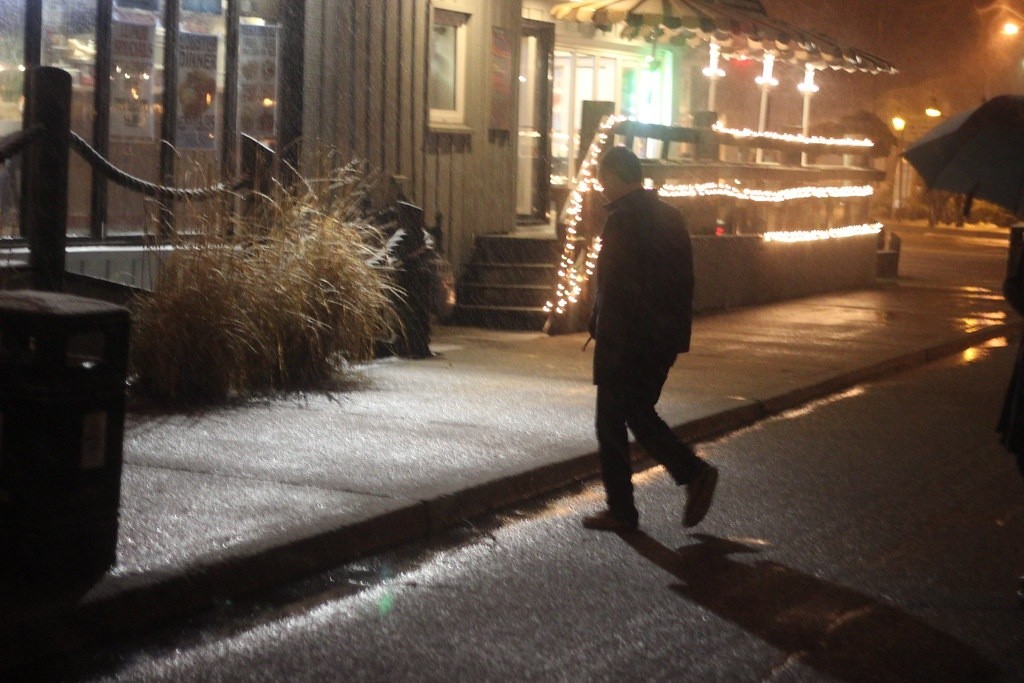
[546,0,894,163]
[904,95,1024,227]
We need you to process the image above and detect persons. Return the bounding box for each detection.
[995,229,1024,469]
[583,141,718,530]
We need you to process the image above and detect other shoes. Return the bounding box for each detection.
[680,462,718,528]
[581,508,638,531]
